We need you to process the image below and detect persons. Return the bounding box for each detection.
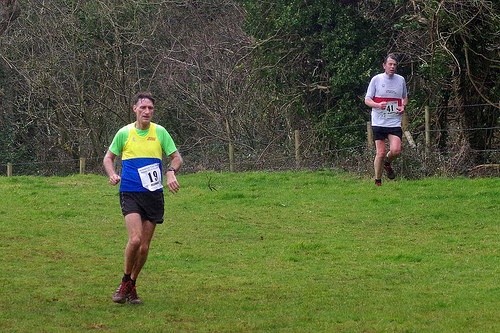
[103,92,184,305]
[365,52,408,187]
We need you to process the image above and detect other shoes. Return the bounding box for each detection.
[127,286,141,304]
[374,179,381,187]
[112,282,128,304]
[384,161,395,179]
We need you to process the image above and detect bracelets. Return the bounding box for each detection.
[164,165,175,173]
